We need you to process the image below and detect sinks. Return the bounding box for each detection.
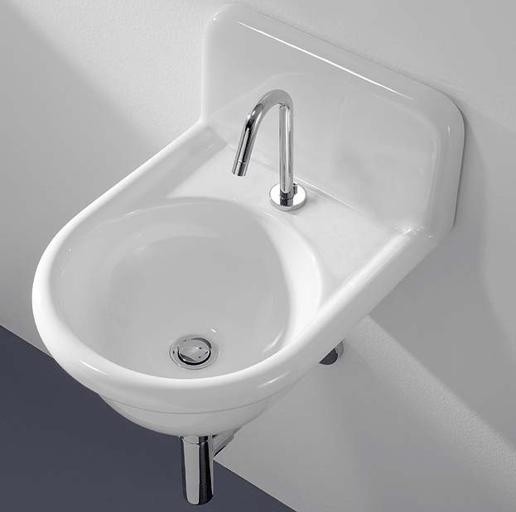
[31,7,463,438]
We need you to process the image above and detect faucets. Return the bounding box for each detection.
[231,89,307,211]
